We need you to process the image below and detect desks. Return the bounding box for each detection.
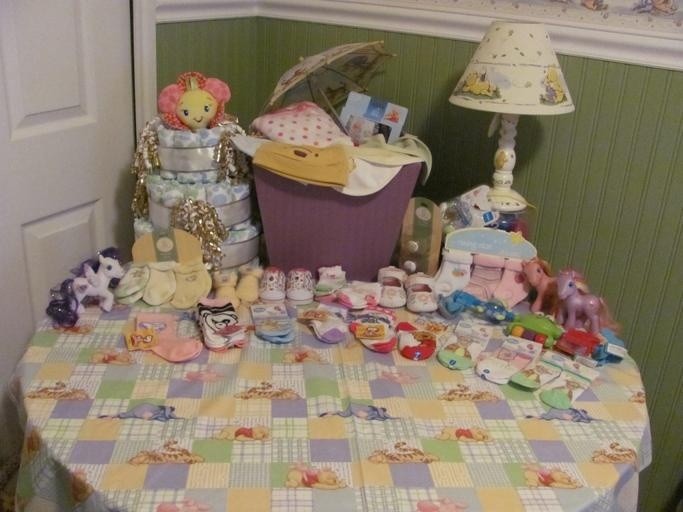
[1,221,656,512]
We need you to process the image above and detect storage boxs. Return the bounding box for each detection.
[248,149,425,284]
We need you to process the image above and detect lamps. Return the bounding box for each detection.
[445,12,581,216]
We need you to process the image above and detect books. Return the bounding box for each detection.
[340,90,408,148]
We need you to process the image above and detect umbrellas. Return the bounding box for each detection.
[259,38,396,138]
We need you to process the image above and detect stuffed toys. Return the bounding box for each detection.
[158,70,232,131]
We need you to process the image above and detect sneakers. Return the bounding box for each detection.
[406,273,441,314]
[236,265,259,302]
[216,267,240,309]
[377,268,409,307]
[288,269,314,301]
[260,267,286,302]
